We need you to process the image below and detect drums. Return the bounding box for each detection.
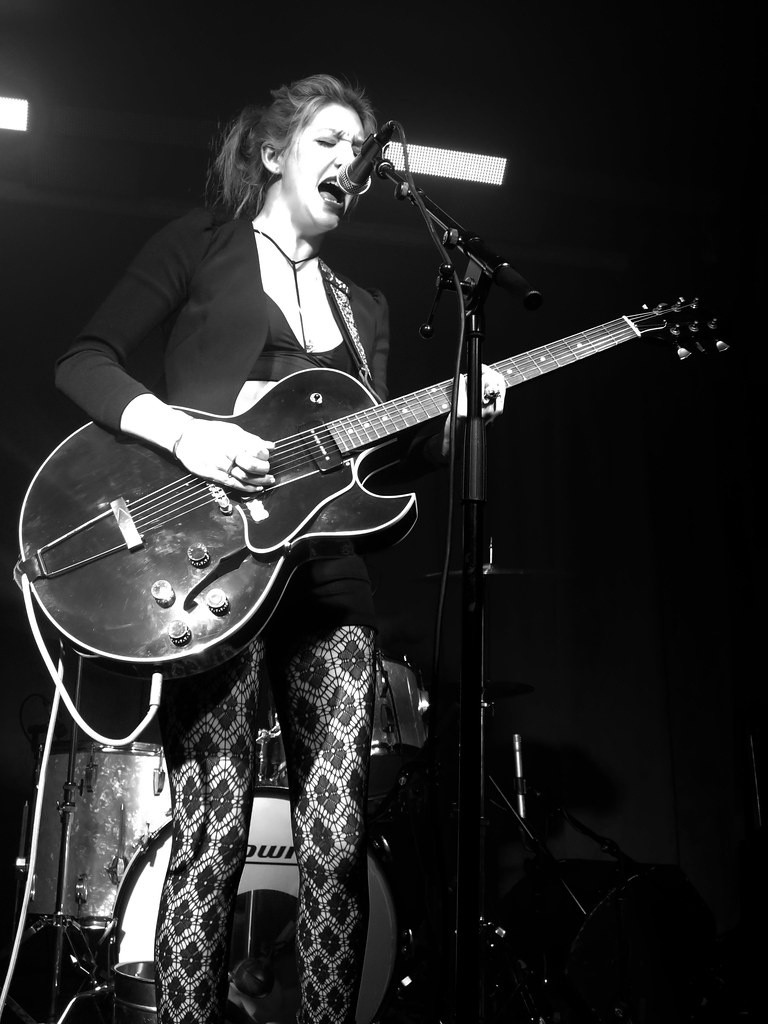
[21,737,177,931]
[109,784,403,1024]
[267,650,432,820]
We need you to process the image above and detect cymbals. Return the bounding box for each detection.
[420,564,552,581]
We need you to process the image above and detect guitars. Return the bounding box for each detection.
[18,292,734,684]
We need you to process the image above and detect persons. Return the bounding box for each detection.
[50,73,505,1024]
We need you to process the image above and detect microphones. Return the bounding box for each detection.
[513,733,526,821]
[336,120,396,195]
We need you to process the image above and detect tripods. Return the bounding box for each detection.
[0,655,113,1024]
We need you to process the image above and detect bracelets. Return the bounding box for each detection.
[171,417,198,461]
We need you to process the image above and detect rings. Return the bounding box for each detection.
[484,384,500,400]
[224,463,237,474]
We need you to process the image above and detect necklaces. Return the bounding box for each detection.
[249,228,319,355]
